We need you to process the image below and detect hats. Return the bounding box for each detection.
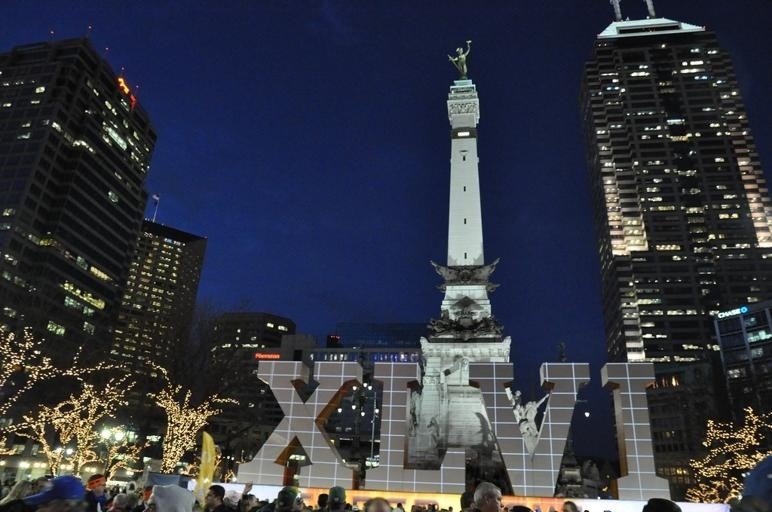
[24,475,85,504]
[326,487,345,504]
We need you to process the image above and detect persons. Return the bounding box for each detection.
[643,498,682,512]
[453,40,474,76]
[597,484,613,499]
[362,481,579,511]
[1,474,357,512]
[730,453,772,511]
